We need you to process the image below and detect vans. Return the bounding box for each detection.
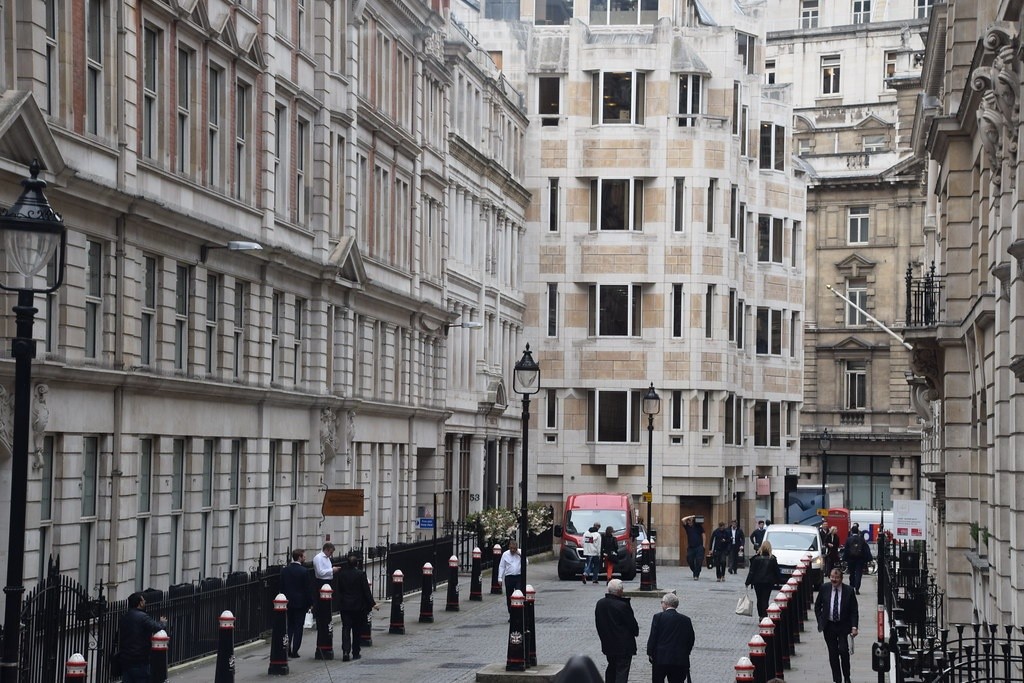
[555,492,641,581]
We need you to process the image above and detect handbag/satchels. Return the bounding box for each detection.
[608,552,620,564]
[110,651,122,676]
[821,545,831,558]
[736,595,754,616]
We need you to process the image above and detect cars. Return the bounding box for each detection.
[635,523,657,568]
[754,524,829,590]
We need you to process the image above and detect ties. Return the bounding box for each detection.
[833,588,839,622]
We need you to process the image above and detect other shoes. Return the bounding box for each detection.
[728,568,733,574]
[343,650,350,661]
[290,652,300,658]
[353,654,362,659]
[722,576,725,581]
[717,577,720,582]
[856,587,860,595]
[694,575,698,580]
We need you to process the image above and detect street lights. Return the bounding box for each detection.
[818,428,832,523]
[0,157,66,683]
[642,381,660,589]
[512,342,542,598]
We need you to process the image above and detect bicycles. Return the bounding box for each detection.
[836,546,878,576]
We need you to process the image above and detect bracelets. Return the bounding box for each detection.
[518,552,520,555]
[852,627,857,630]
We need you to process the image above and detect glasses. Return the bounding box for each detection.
[661,601,666,604]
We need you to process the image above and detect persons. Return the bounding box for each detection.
[681,515,770,582]
[819,520,866,595]
[497,540,529,623]
[280,549,313,658]
[594,578,640,683]
[745,541,781,622]
[314,543,342,629]
[814,568,859,683]
[647,593,695,683]
[335,556,379,662]
[582,522,602,585]
[601,526,618,586]
[118,592,168,683]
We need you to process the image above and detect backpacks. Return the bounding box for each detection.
[847,537,863,560]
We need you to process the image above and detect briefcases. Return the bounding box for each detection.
[706,554,713,569]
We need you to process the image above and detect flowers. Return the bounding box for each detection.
[469,499,553,539]
